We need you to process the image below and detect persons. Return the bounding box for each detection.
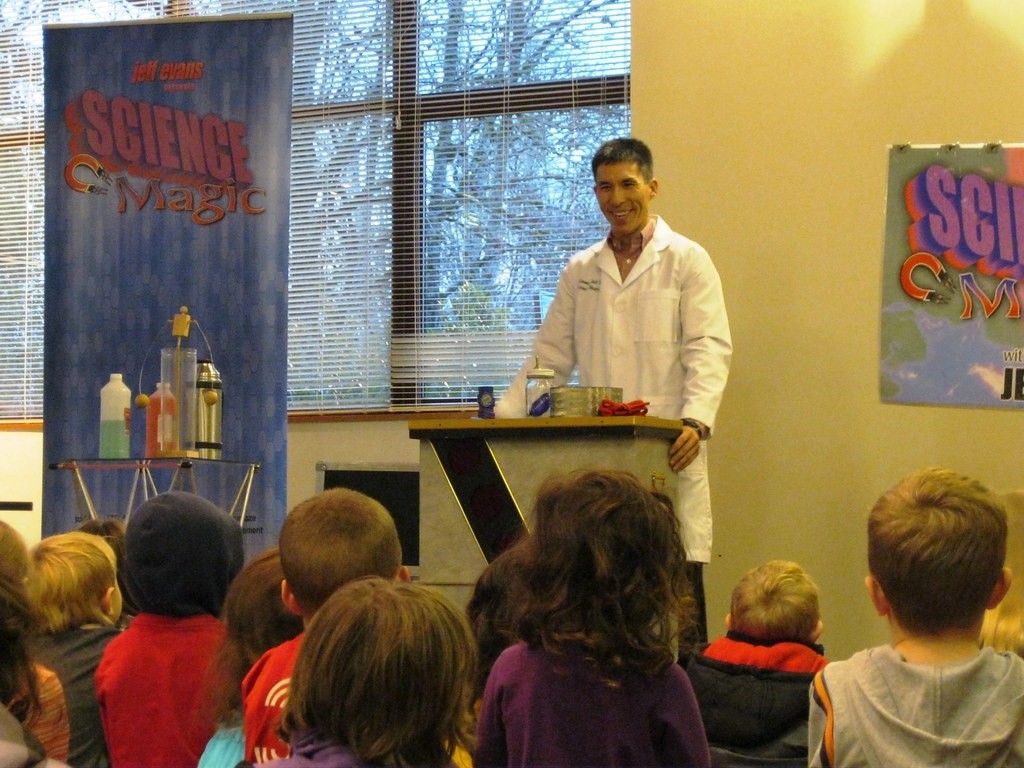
[803,467,1024,767]
[25,528,125,768]
[238,482,416,764]
[677,558,823,767]
[469,465,712,767]
[91,490,236,768]
[493,136,733,581]
[980,489,1022,659]
[249,573,470,768]
[75,515,138,629]
[0,524,71,768]
[477,538,538,679]
[192,549,303,767]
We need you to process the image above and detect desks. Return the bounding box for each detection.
[50,458,259,528]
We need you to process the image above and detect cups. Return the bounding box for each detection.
[160,348,197,453]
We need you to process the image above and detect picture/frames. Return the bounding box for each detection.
[878,145,1024,408]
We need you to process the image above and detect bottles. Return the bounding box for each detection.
[568,363,590,388]
[146,382,174,457]
[478,385,494,419]
[100,374,133,460]
[525,367,554,419]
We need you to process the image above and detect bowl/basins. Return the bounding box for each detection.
[550,387,623,419]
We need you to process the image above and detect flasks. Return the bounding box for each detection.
[186,360,225,461]
